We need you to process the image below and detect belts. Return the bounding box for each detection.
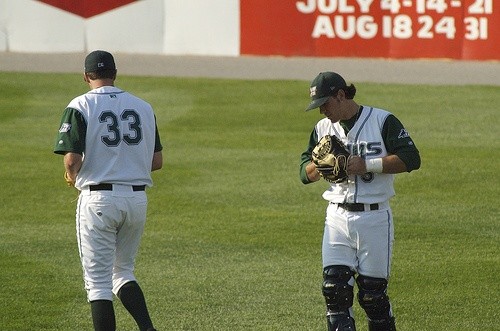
[89,183,146,192]
[330,202,378,212]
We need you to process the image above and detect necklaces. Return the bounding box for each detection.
[355,105,360,122]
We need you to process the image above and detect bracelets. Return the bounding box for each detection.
[365,158,383,174]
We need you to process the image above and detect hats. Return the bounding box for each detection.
[85,50,116,74]
[305,72,347,112]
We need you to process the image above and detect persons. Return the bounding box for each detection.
[56,50,163,331]
[298,71,421,331]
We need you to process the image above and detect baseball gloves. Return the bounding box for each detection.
[312,135,352,183]
